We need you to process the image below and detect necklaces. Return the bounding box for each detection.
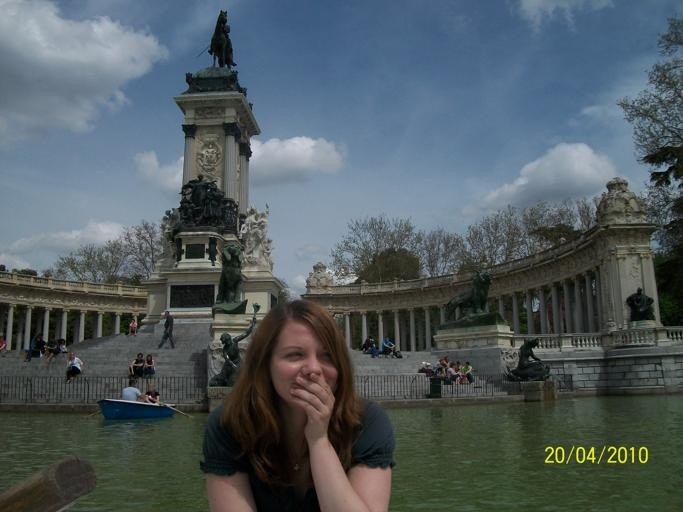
[276,438,306,471]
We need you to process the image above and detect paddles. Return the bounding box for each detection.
[147,395,192,418]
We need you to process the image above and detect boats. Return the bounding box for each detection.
[96,399,177,420]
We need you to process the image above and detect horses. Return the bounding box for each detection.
[210,10,230,69]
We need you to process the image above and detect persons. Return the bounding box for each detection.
[200,299,395,511]
[0,336,6,351]
[627,287,655,321]
[121,353,160,403]
[365,336,373,352]
[209,317,255,387]
[384,337,397,358]
[161,175,224,240]
[421,356,474,385]
[65,353,83,384]
[514,338,550,380]
[158,311,176,348]
[128,319,137,335]
[24,336,67,362]
